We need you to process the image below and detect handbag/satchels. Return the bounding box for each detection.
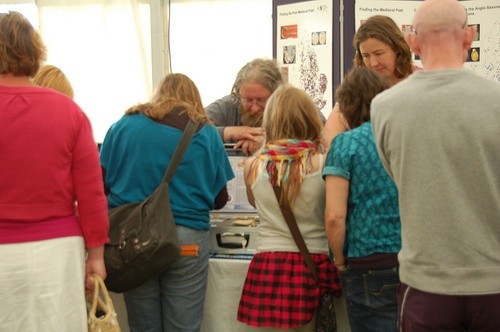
[314,293,340,332]
[84,275,122,332]
[98,182,180,295]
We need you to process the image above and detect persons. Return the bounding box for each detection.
[322,66,401,332]
[236,85,343,332]
[370,0,500,332]
[29,64,75,101]
[98,73,236,332]
[319,15,424,155]
[202,58,327,157]
[0,10,109,332]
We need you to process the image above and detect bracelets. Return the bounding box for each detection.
[333,256,345,266]
